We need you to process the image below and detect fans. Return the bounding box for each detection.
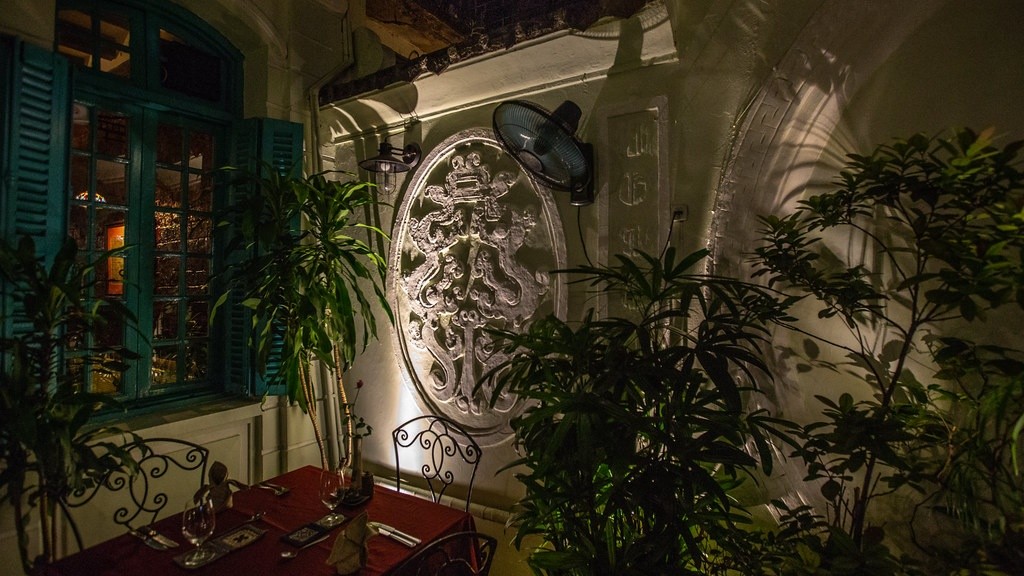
[491,99,594,207]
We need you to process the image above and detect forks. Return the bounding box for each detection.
[255,484,291,496]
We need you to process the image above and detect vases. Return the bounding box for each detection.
[343,437,365,504]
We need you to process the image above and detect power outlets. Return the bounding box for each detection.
[670,204,688,222]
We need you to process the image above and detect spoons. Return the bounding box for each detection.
[280,534,330,559]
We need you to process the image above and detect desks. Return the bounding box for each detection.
[35,466,486,576]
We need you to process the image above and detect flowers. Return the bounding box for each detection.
[339,380,373,438]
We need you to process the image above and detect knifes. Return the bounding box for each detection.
[368,524,416,548]
[143,526,179,548]
[369,521,422,544]
[129,529,167,552]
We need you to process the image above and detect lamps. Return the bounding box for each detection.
[359,142,421,191]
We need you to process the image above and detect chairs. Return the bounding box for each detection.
[391,531,497,576]
[50,438,210,552]
[392,416,482,511]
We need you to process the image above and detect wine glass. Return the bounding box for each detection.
[320,471,345,527]
[182,499,215,566]
